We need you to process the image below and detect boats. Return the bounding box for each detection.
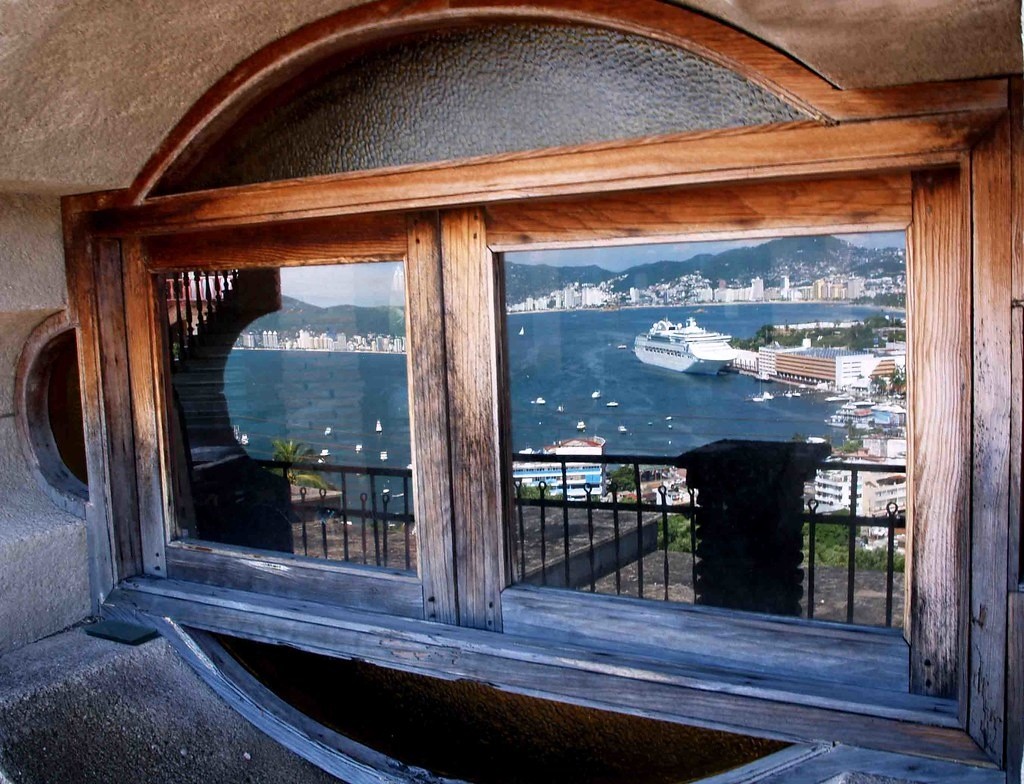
[513,447,602,503]
[634,316,738,377]
